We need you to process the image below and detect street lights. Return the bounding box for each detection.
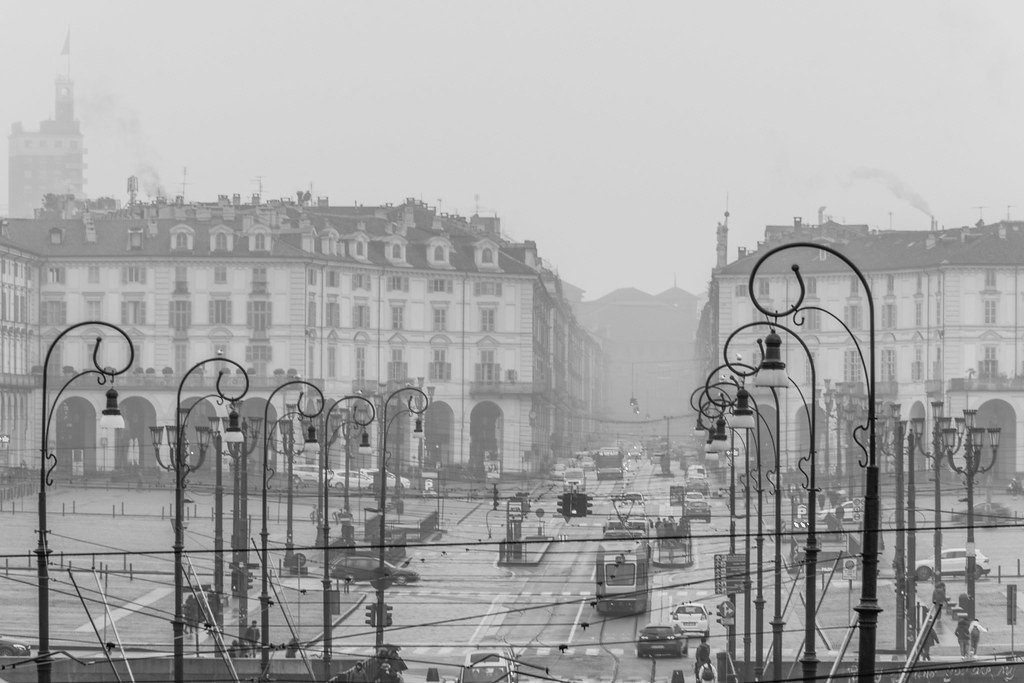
[34,320,428,683]
[822,378,1001,622]
[690,243,884,683]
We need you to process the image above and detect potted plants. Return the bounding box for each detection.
[162,367,174,377]
[273,368,285,375]
[247,368,256,374]
[287,368,297,376]
[220,366,231,375]
[62,366,75,377]
[236,367,246,377]
[146,368,155,376]
[189,366,203,376]
[133,367,145,376]
[31,365,43,375]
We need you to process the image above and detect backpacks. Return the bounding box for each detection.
[971,626,980,639]
[699,646,709,661]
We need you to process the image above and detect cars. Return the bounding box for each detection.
[681,463,711,523]
[332,468,374,492]
[329,557,420,586]
[953,502,1009,522]
[550,440,644,492]
[362,469,409,490]
[0,638,31,665]
[818,500,853,520]
[637,603,713,658]
[454,635,521,683]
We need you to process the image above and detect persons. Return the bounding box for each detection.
[349,647,409,683]
[782,483,846,529]
[915,583,988,662]
[696,637,718,683]
[185,589,299,658]
[1010,477,1019,499]
[655,516,688,544]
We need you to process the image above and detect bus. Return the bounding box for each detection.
[595,498,654,617]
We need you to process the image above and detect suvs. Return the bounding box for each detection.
[906,549,991,581]
[292,466,329,487]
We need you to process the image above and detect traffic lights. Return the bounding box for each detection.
[206,596,216,624]
[231,570,239,591]
[382,603,392,628]
[716,604,721,624]
[576,492,592,517]
[247,571,254,590]
[556,492,572,517]
[629,399,640,415]
[365,603,375,627]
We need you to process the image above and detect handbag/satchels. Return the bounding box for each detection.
[702,665,714,680]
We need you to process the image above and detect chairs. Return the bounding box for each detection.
[694,607,702,612]
